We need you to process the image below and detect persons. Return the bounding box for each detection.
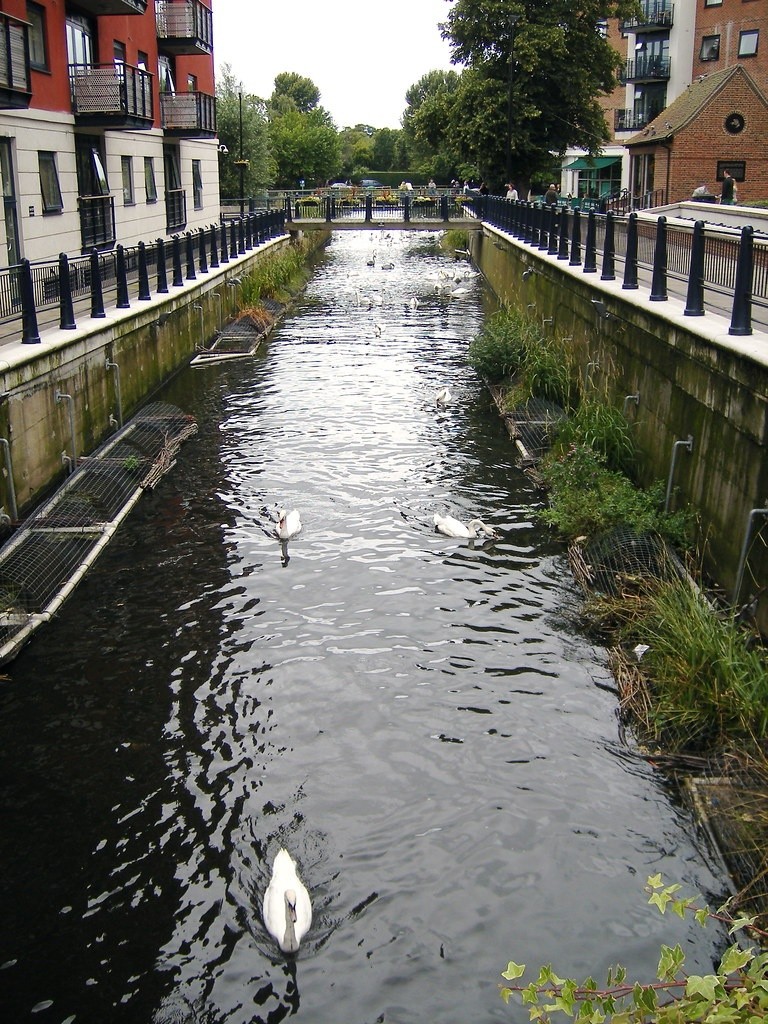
[463,180,469,193]
[506,183,518,202]
[720,170,738,205]
[545,184,560,206]
[429,179,436,188]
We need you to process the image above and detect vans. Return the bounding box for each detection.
[359,180,384,189]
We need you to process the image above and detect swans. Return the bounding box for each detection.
[262,847,312,954]
[276,509,302,540]
[338,229,481,334]
[436,387,453,404]
[433,514,501,540]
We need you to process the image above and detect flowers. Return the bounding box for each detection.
[335,197,361,206]
[417,196,431,201]
[455,196,473,202]
[376,195,399,202]
[294,196,322,205]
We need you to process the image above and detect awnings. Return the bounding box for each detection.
[561,157,620,169]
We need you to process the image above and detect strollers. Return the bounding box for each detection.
[691,185,720,204]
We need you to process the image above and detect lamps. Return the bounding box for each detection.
[217,145,228,154]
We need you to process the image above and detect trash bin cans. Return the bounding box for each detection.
[297,197,323,219]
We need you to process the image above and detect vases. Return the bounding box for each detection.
[340,201,359,206]
[302,201,319,208]
[376,200,398,208]
[413,198,435,208]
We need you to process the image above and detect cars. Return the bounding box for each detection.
[331,182,354,189]
